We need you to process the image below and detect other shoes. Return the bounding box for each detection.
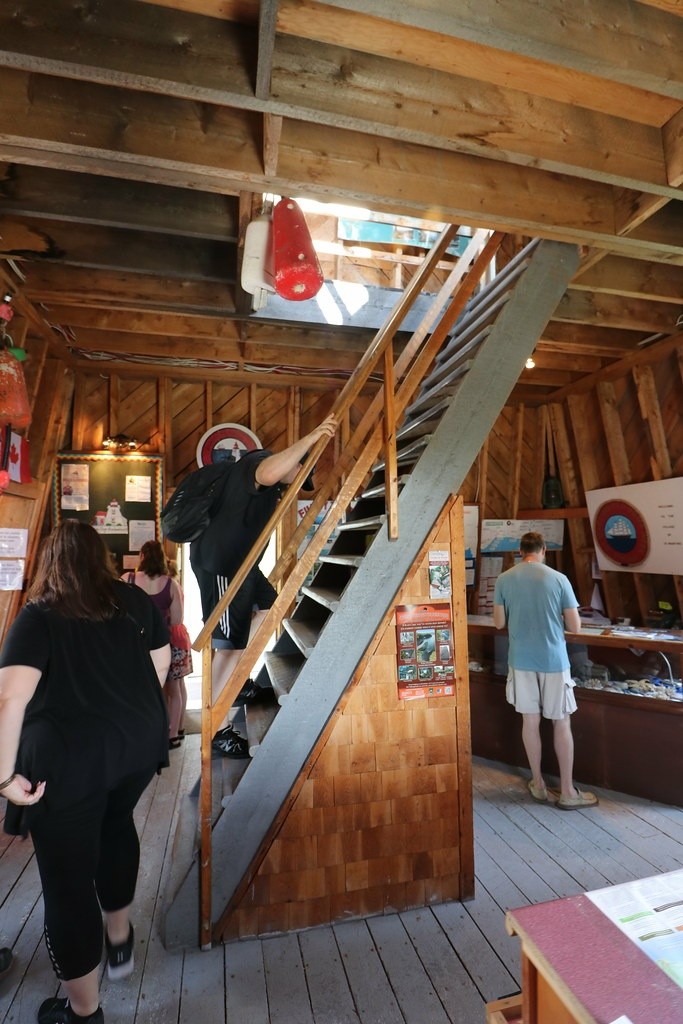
[166,728,186,751]
[0,947,14,975]
[555,785,600,810]
[526,776,549,803]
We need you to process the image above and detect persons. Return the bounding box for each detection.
[493,533,599,809]
[0,521,170,1024]
[190,413,337,761]
[164,558,192,749]
[116,542,183,691]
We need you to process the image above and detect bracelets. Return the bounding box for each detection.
[0,773,15,790]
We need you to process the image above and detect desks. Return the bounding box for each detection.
[485,863,683,1024]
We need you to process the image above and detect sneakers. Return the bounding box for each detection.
[104,921,135,982]
[37,995,104,1024]
[211,724,253,759]
[230,677,276,706]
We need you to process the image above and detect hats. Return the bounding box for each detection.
[299,450,317,491]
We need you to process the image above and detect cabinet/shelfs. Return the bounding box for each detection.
[467,615,683,808]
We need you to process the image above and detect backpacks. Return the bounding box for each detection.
[160,446,273,544]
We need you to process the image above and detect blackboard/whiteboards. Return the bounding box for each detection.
[50,450,166,580]
